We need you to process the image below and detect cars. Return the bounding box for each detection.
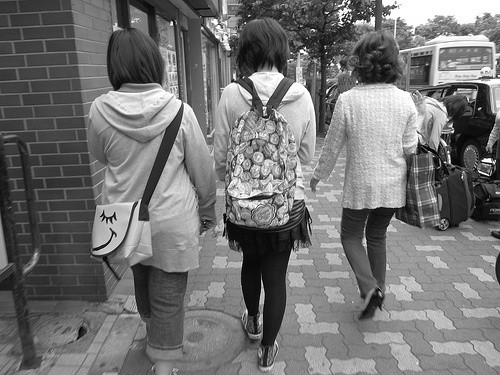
[324,82,339,124]
[417,67,500,148]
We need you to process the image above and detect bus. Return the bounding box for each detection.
[399,34,496,92]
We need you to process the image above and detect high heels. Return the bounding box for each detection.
[359,286,383,319]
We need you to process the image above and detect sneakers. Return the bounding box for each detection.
[241,311,261,339]
[258,340,279,370]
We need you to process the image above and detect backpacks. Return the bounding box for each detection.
[224,77,299,228]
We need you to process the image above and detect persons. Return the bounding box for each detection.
[88,27,216,375]
[310,29,417,318]
[416,94,469,159]
[214,17,316,373]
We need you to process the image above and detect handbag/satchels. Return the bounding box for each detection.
[91,199,153,267]
[419,144,447,180]
[472,183,500,221]
[396,137,441,228]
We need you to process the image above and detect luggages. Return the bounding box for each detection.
[435,162,476,230]
